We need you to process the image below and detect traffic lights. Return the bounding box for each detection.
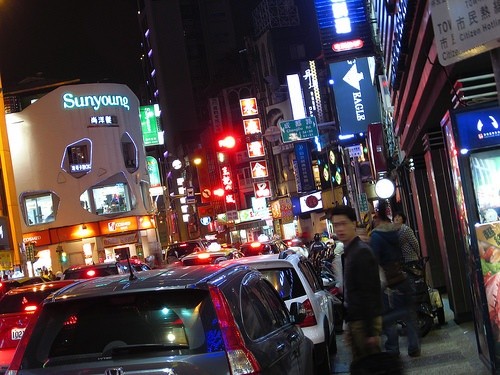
[32,250,40,263]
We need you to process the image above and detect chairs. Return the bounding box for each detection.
[48,327,109,357]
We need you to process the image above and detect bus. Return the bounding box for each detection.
[162,238,212,257]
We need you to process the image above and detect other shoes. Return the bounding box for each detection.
[408,350,419,357]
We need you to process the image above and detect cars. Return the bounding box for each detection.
[181,234,290,265]
[317,261,341,299]
[0,277,84,367]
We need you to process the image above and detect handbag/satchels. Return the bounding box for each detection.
[409,276,432,305]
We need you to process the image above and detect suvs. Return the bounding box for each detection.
[7,253,319,375]
[60,260,152,280]
[213,248,339,374]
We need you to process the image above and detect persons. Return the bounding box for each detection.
[42,269,58,282]
[0,277,2,282]
[3,275,8,281]
[389,212,422,269]
[318,204,388,373]
[367,206,421,359]
